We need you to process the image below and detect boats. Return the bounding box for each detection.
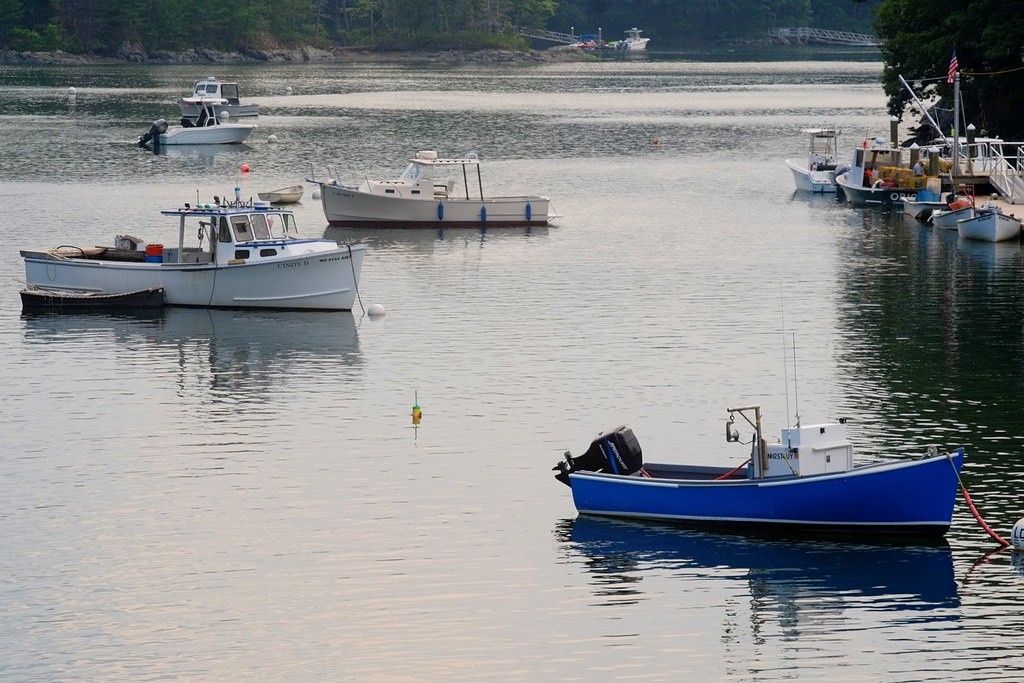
[786,128,851,192]
[19,181,365,311]
[27,312,360,364]
[569,514,961,609]
[929,72,1023,205]
[902,189,949,218]
[570,39,613,49]
[957,194,1022,242]
[176,76,259,116]
[150,102,257,143]
[835,115,941,206]
[613,27,650,48]
[932,199,974,228]
[569,283,965,533]
[305,151,562,227]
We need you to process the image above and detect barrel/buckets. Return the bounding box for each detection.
[144,244,163,263]
[949,200,968,211]
[915,190,939,202]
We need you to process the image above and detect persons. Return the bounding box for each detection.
[913,159,925,177]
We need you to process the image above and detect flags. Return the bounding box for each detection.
[947,51,958,83]
